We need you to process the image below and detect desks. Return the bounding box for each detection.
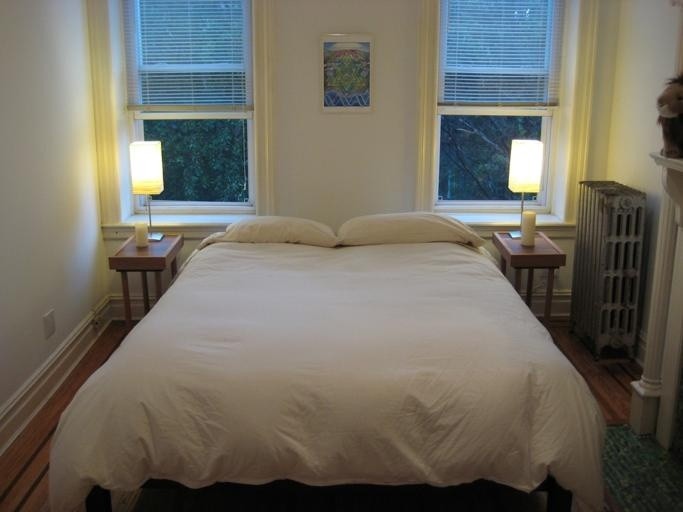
[492,230,566,330]
[107,231,184,336]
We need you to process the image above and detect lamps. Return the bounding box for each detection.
[129,139,164,241]
[507,139,544,240]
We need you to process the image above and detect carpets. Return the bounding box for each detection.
[572,423,682,511]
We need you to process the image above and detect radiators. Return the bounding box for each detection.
[567,181,646,363]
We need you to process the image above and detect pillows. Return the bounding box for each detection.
[214,211,486,247]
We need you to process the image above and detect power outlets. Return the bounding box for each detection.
[42,309,57,339]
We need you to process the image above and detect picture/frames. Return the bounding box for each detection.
[318,32,375,114]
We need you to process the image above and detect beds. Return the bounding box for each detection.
[81,227,572,512]
